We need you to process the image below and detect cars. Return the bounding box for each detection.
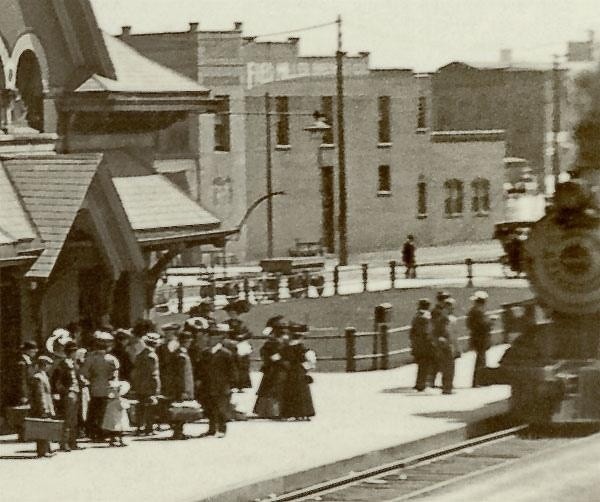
[289,242,322,257]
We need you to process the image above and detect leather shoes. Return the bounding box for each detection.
[33,427,226,459]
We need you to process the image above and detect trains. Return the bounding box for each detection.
[475,166,600,425]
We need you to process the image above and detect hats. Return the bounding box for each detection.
[103,379,131,400]
[20,299,310,363]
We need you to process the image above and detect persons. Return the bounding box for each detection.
[467,289,495,387]
[427,290,450,388]
[401,234,417,278]
[435,298,458,395]
[511,229,526,278]
[409,297,433,390]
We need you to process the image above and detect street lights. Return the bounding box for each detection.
[204,92,327,259]
